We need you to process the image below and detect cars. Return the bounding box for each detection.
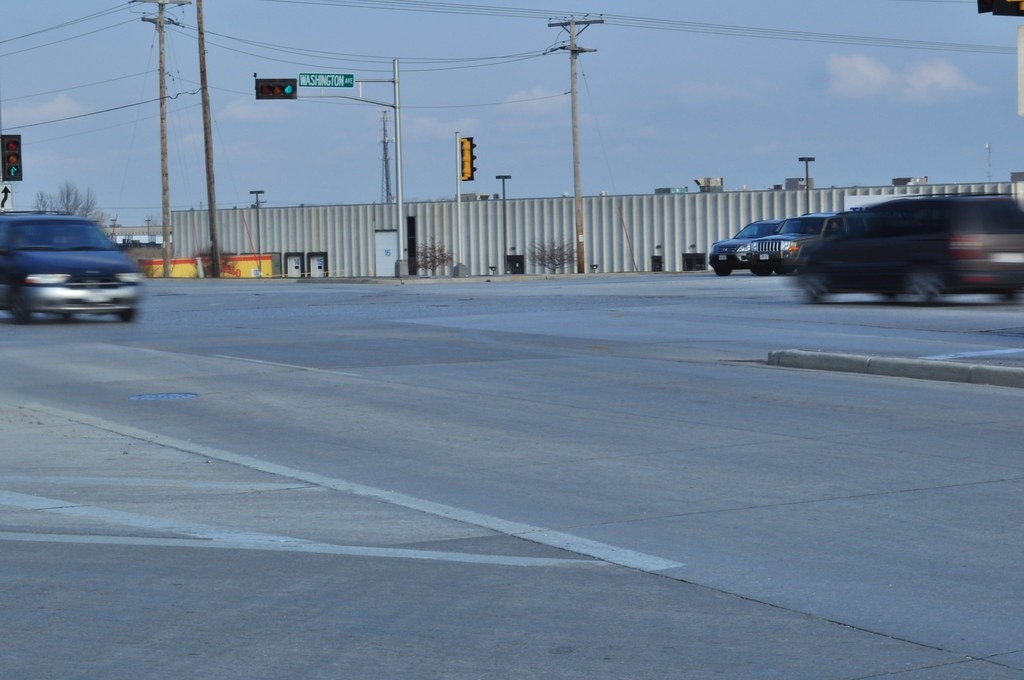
[0,209,144,325]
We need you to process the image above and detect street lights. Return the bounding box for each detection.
[799,156,815,215]
[496,175,512,274]
[250,190,264,274]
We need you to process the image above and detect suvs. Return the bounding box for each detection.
[746,212,837,277]
[709,219,787,278]
[798,193,1024,308]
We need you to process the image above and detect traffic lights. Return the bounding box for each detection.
[255,78,297,100]
[2,134,24,182]
[460,137,477,182]
